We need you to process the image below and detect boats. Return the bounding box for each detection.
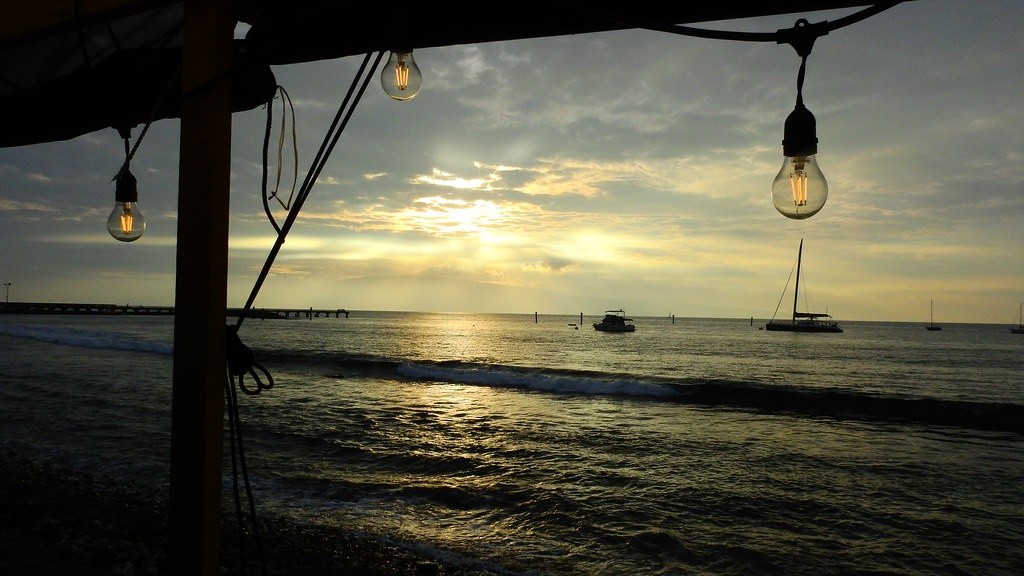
[592,314,636,333]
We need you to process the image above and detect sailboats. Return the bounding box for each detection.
[1009,303,1024,334]
[925,299,942,330]
[766,239,844,333]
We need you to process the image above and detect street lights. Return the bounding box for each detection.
[4,283,12,302]
